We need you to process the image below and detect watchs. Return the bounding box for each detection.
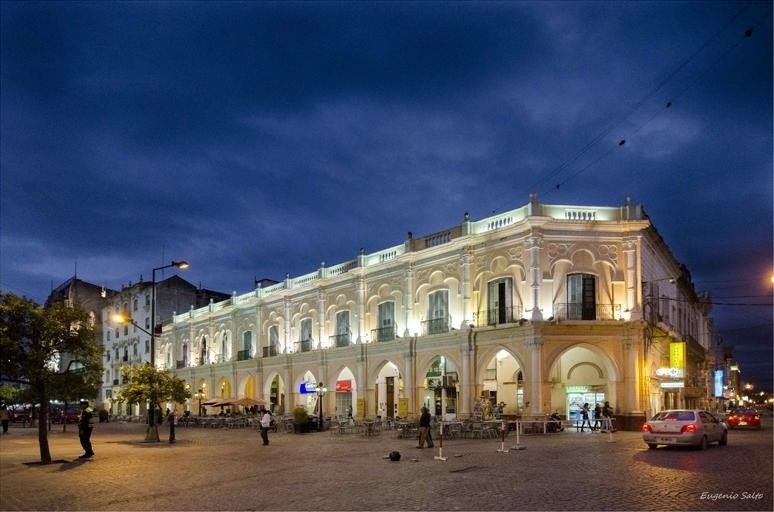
[545,412,563,432]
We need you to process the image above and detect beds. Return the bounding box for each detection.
[200,397,275,416]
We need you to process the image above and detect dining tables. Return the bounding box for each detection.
[291,406,308,434]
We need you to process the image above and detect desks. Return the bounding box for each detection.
[195,389,206,416]
[113,261,188,443]
[316,382,327,430]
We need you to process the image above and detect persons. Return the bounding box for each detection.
[600,401,615,433]
[607,404,618,432]
[593,402,602,430]
[0,405,11,433]
[258,407,271,445]
[6,402,80,427]
[577,403,595,432]
[97,409,109,423]
[75,401,96,459]
[418,408,433,445]
[415,406,435,449]
[165,407,171,417]
[346,404,355,425]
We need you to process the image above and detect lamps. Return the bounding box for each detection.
[664,412,691,421]
[311,416,509,439]
[108,413,291,432]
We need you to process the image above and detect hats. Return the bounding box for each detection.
[7,400,95,424]
[642,410,728,450]
[726,406,760,429]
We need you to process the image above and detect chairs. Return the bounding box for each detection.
[79,451,95,459]
